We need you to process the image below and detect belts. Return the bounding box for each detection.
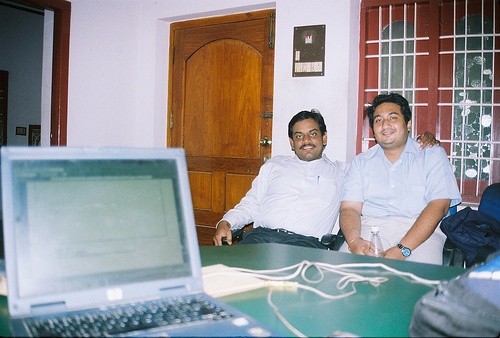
[270,228,296,235]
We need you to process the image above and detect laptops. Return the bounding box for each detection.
[0,145,278,338]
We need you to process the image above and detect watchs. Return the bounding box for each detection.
[397,243,412,257]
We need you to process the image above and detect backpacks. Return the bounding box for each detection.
[441,206,500,265]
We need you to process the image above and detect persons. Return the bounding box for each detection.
[213,111,441,246]
[337,92,463,266]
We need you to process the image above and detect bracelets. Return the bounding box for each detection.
[347,237,362,252]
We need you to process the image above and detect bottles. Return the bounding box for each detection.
[366,227,385,257]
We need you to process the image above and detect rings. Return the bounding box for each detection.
[432,138,436,142]
[435,140,440,143]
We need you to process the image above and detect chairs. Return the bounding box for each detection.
[453,184,500,269]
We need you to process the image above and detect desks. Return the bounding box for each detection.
[0,243,468,338]
